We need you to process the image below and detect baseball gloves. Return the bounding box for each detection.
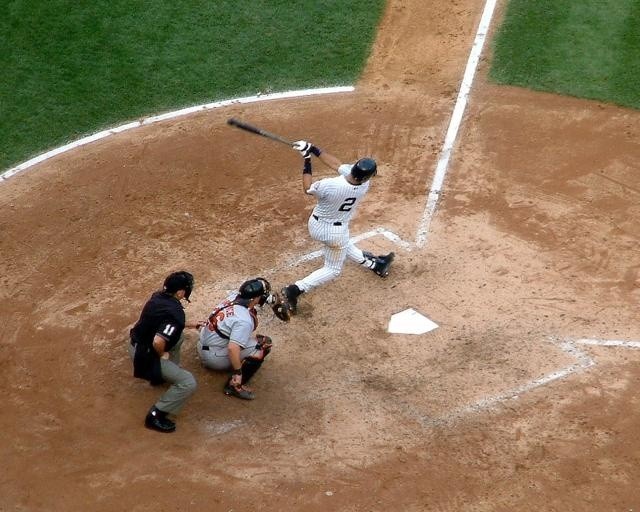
[270,292,293,323]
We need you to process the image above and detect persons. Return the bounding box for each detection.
[130,271,207,433]
[197,277,290,400]
[281,140,395,315]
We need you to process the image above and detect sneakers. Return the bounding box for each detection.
[281,283,302,316]
[224,381,255,400]
[144,410,176,433]
[373,250,395,277]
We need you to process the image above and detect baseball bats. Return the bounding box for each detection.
[226,118,296,147]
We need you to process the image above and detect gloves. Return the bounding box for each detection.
[292,140,323,175]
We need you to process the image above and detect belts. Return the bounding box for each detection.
[313,215,343,227]
[201,344,209,350]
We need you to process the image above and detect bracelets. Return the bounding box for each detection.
[233,368,242,376]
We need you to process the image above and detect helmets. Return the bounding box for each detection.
[350,157,377,184]
[162,270,193,300]
[238,277,265,300]
